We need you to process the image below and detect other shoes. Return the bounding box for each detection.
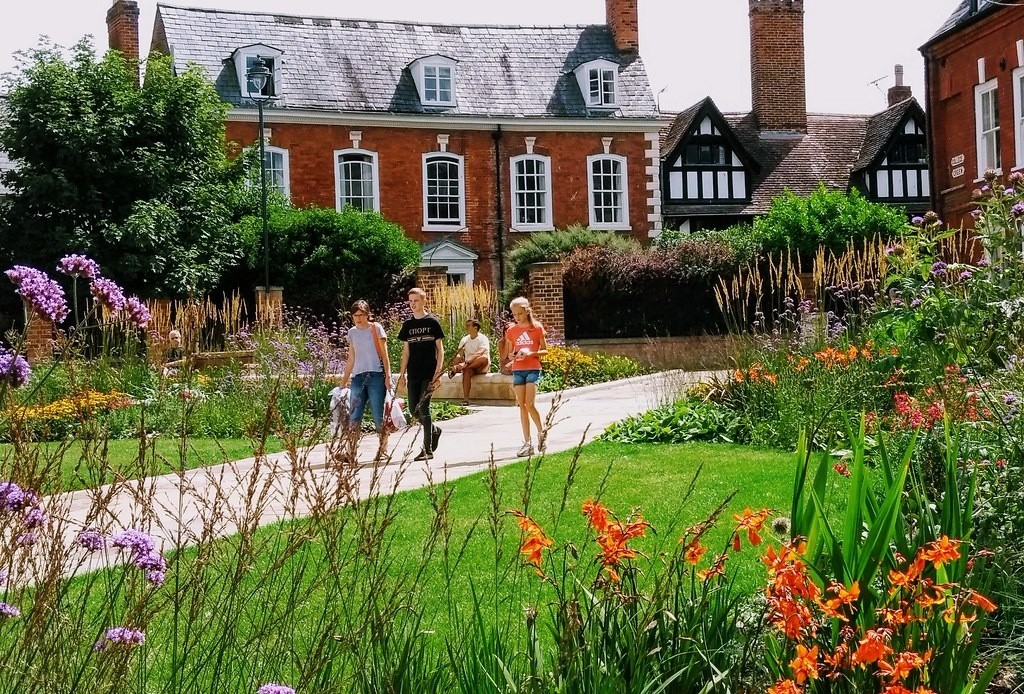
[460,401,469,406]
[335,453,351,464]
[449,371,455,379]
[376,450,388,460]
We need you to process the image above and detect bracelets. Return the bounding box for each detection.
[467,362,470,365]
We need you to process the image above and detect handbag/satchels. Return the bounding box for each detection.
[328,387,351,437]
[379,357,395,384]
[383,388,407,433]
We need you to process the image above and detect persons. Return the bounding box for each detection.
[506,297,548,457]
[334,300,393,463]
[398,288,442,461]
[499,322,516,375]
[162,330,191,379]
[448,320,490,406]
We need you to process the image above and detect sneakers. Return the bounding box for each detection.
[516,441,534,456]
[414,447,433,461]
[429,427,442,452]
[538,432,547,452]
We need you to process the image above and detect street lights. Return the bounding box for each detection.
[244,52,282,290]
